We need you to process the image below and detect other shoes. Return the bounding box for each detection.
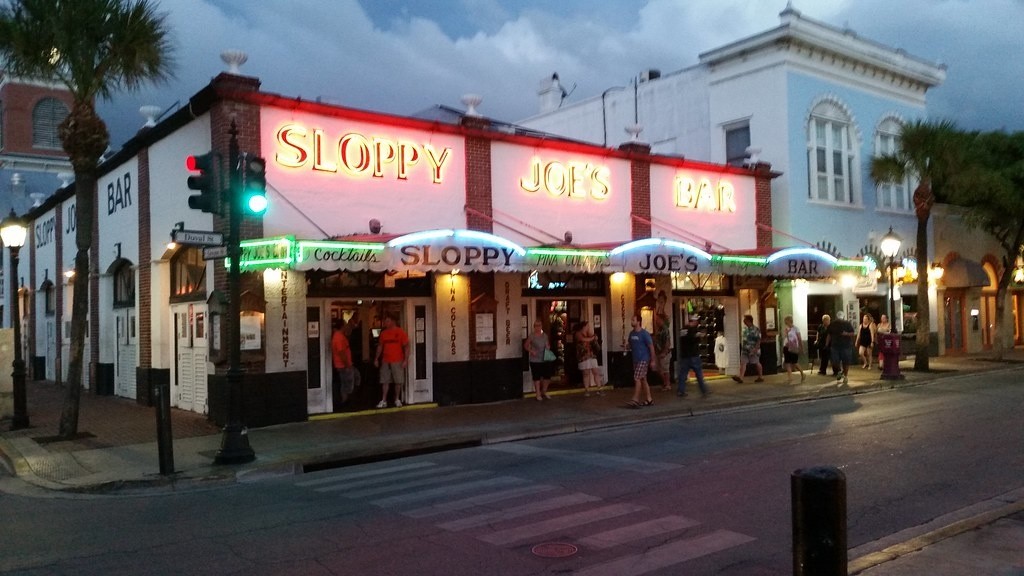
[733,376,743,384]
[583,392,591,397]
[541,394,551,399]
[536,396,543,401]
[785,378,794,382]
[862,364,867,368]
[801,375,806,383]
[661,386,672,393]
[818,371,826,374]
[677,393,687,398]
[843,377,848,383]
[878,366,884,370]
[342,401,355,410]
[596,391,607,396]
[703,389,712,396]
[868,363,874,370]
[754,377,764,382]
[836,371,842,380]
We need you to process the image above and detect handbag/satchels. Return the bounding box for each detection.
[544,348,557,362]
[592,335,600,355]
[788,346,800,354]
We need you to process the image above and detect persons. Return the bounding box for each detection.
[677,326,712,395]
[732,314,764,383]
[876,312,891,370]
[714,332,730,373]
[652,314,672,391]
[854,313,877,370]
[524,320,555,401]
[782,316,806,382]
[331,318,363,402]
[623,314,656,407]
[374,316,410,408]
[814,314,837,375]
[573,320,606,397]
[824,310,855,383]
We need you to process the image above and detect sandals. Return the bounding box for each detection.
[625,400,639,408]
[640,399,655,407]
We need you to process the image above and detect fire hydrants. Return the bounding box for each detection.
[873,331,907,381]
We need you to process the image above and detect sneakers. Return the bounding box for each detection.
[376,400,387,408]
[395,400,402,407]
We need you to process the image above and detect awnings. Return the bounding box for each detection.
[224,227,871,283]
[943,258,991,288]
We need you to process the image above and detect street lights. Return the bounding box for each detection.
[879,224,902,333]
[0,205,32,431]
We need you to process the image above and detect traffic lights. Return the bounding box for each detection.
[186,150,222,215]
[234,153,268,219]
